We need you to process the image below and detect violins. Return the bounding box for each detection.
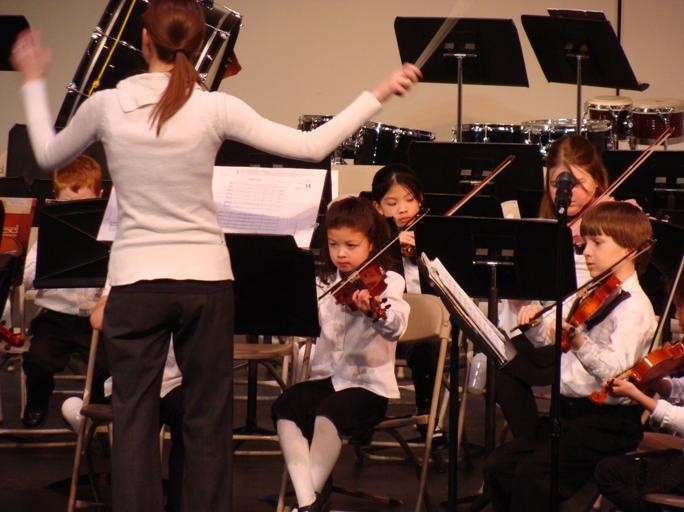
[333,258,391,323]
[591,340,683,408]
[556,272,621,353]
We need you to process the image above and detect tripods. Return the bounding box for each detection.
[255,264,541,512]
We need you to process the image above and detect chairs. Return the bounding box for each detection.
[17,280,684,512]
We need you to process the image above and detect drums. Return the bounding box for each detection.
[298,114,356,160]
[584,98,635,142]
[56,0,241,136]
[628,107,682,149]
[354,121,431,165]
[455,119,614,152]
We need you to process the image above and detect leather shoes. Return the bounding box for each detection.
[23,413,43,429]
[420,427,449,450]
[290,491,332,512]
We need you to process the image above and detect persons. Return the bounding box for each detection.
[269,195,414,512]
[480,200,664,512]
[12,0,423,512]
[600,366,684,439]
[353,162,448,448]
[487,128,644,437]
[0,154,113,430]
[96,322,185,512]
